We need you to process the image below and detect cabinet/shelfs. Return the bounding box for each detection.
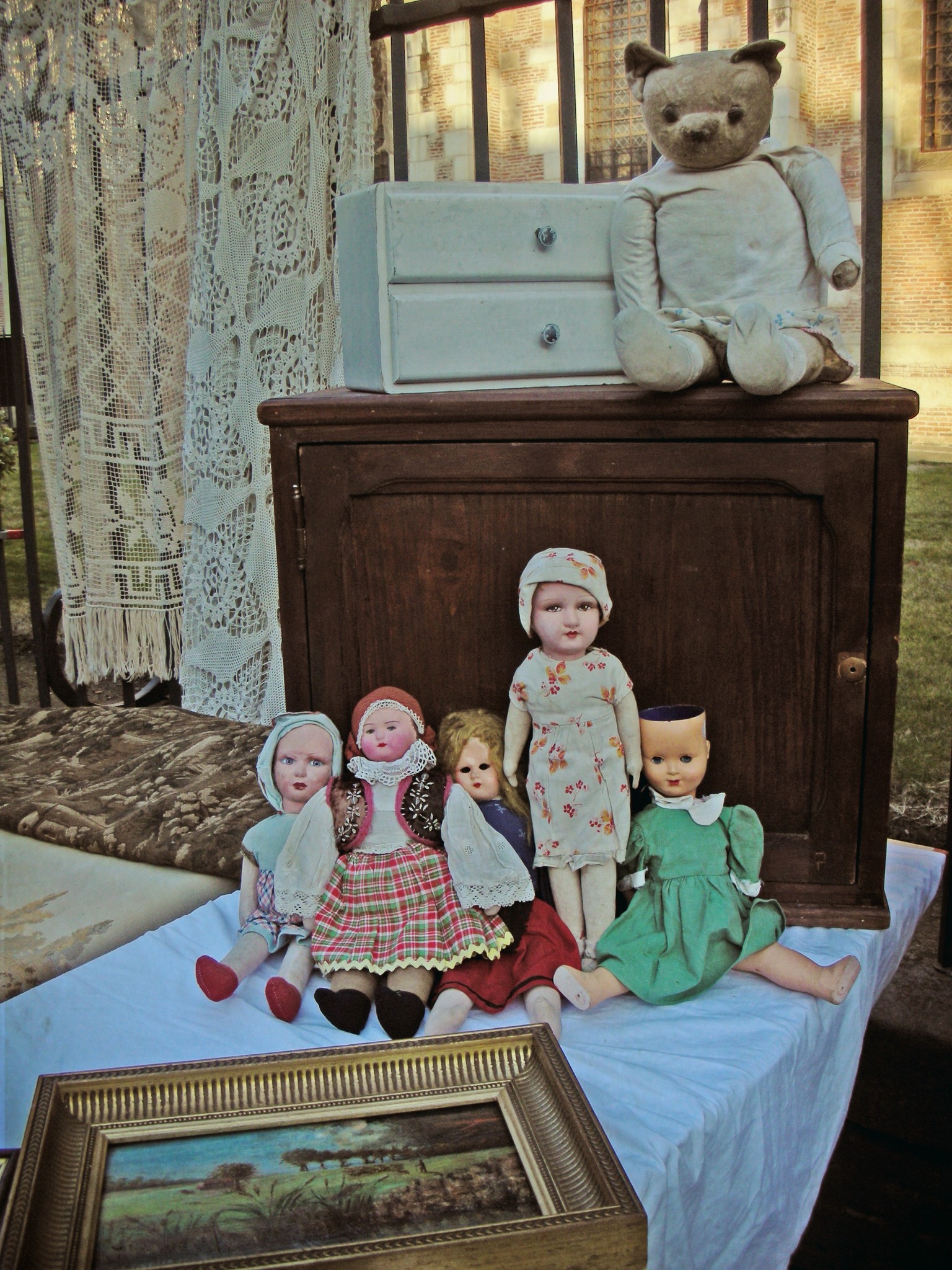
[255,371,926,936]
[328,178,640,392]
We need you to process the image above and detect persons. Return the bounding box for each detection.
[194,548,861,1040]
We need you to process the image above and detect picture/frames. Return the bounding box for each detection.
[0,1019,651,1270]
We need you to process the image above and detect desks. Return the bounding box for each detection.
[0,835,952,1270]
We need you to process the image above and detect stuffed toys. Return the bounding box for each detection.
[610,40,863,395]
